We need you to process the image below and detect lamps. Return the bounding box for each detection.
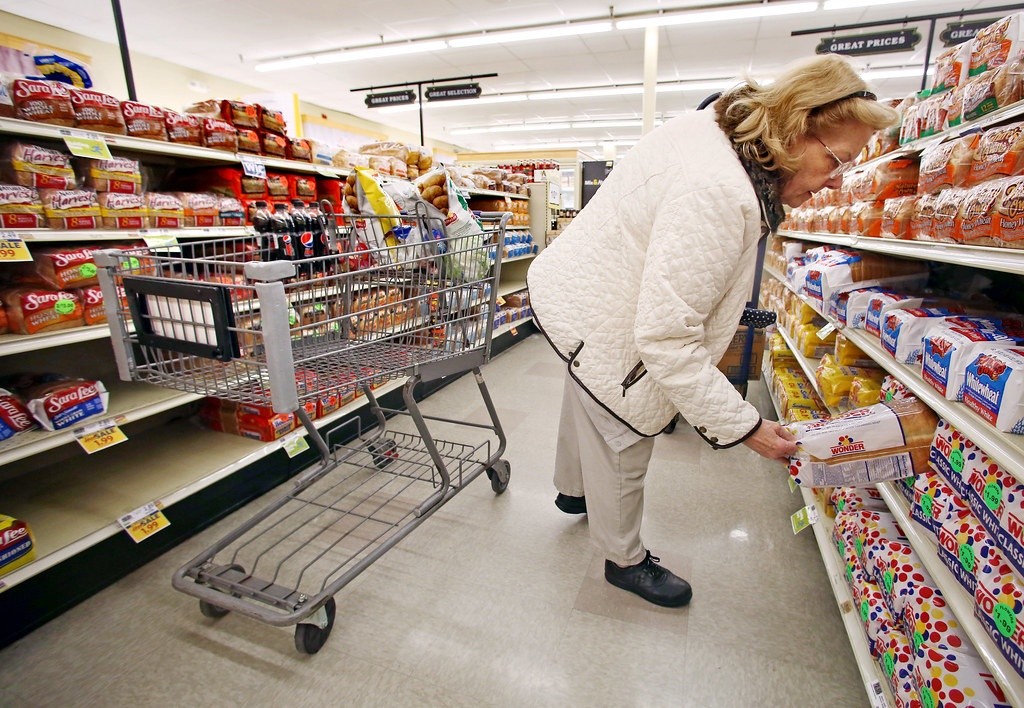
[214,1,938,149]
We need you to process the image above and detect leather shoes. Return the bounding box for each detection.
[555,492,587,514]
[605,549,692,608]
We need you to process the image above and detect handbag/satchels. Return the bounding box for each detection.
[341,163,490,285]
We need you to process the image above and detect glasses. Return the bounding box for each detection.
[807,131,853,180]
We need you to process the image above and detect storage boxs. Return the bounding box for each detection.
[716,325,766,380]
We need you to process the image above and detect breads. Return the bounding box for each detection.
[780,13,1024,251]
[0,73,529,440]
[756,279,938,515]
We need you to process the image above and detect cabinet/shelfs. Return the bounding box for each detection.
[523,180,561,253]
[2,80,535,653]
[762,92,1022,707]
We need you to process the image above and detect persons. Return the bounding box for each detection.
[526,53,900,608]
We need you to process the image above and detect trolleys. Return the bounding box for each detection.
[91,212,511,655]
[660,230,770,436]
